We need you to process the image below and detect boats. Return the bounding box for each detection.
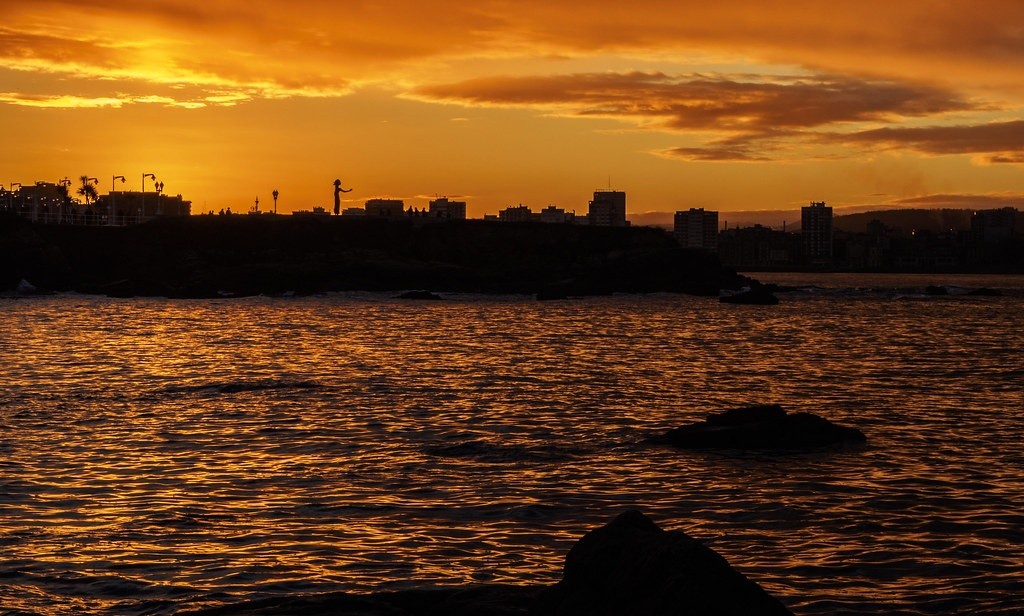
[400,289,430,299]
[719,288,779,305]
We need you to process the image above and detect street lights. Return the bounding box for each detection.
[40,193,46,222]
[53,197,59,222]
[27,193,33,223]
[1,191,7,213]
[142,174,155,224]
[83,178,99,225]
[155,182,163,213]
[11,183,23,210]
[273,190,279,214]
[35,183,46,218]
[113,176,126,225]
[58,180,71,224]
